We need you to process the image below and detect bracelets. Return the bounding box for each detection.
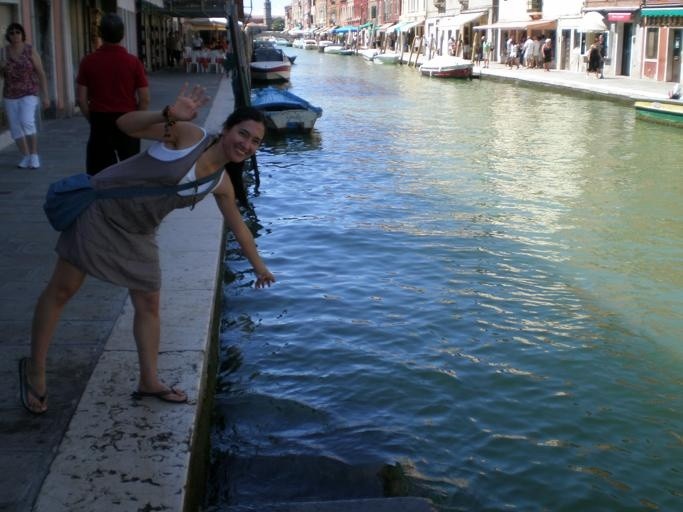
[163,105,176,138]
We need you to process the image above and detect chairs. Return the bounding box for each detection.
[181,45,226,74]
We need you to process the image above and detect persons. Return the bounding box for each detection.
[19,81,277,412]
[76,13,151,177]
[586,34,607,79]
[0,22,50,169]
[166,30,232,72]
[337,34,555,70]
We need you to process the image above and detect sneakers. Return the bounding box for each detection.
[17,154,40,168]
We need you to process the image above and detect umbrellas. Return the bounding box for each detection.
[313,25,361,33]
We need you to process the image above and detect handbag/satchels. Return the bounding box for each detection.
[42,173,98,232]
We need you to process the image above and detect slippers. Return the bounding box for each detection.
[17,357,51,414]
[137,384,189,404]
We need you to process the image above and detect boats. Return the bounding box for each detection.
[634,101,683,129]
[247,27,480,138]
